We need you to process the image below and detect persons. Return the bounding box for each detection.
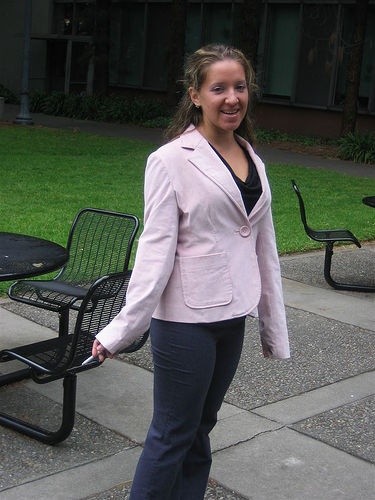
[91,42,291,500]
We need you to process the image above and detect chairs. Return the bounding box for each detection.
[0,270,154,447]
[291,179,375,293]
[0,206,140,385]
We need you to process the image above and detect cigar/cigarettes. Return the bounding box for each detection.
[81,355,101,367]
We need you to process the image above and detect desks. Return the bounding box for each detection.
[361,196,375,210]
[0,231,69,282]
